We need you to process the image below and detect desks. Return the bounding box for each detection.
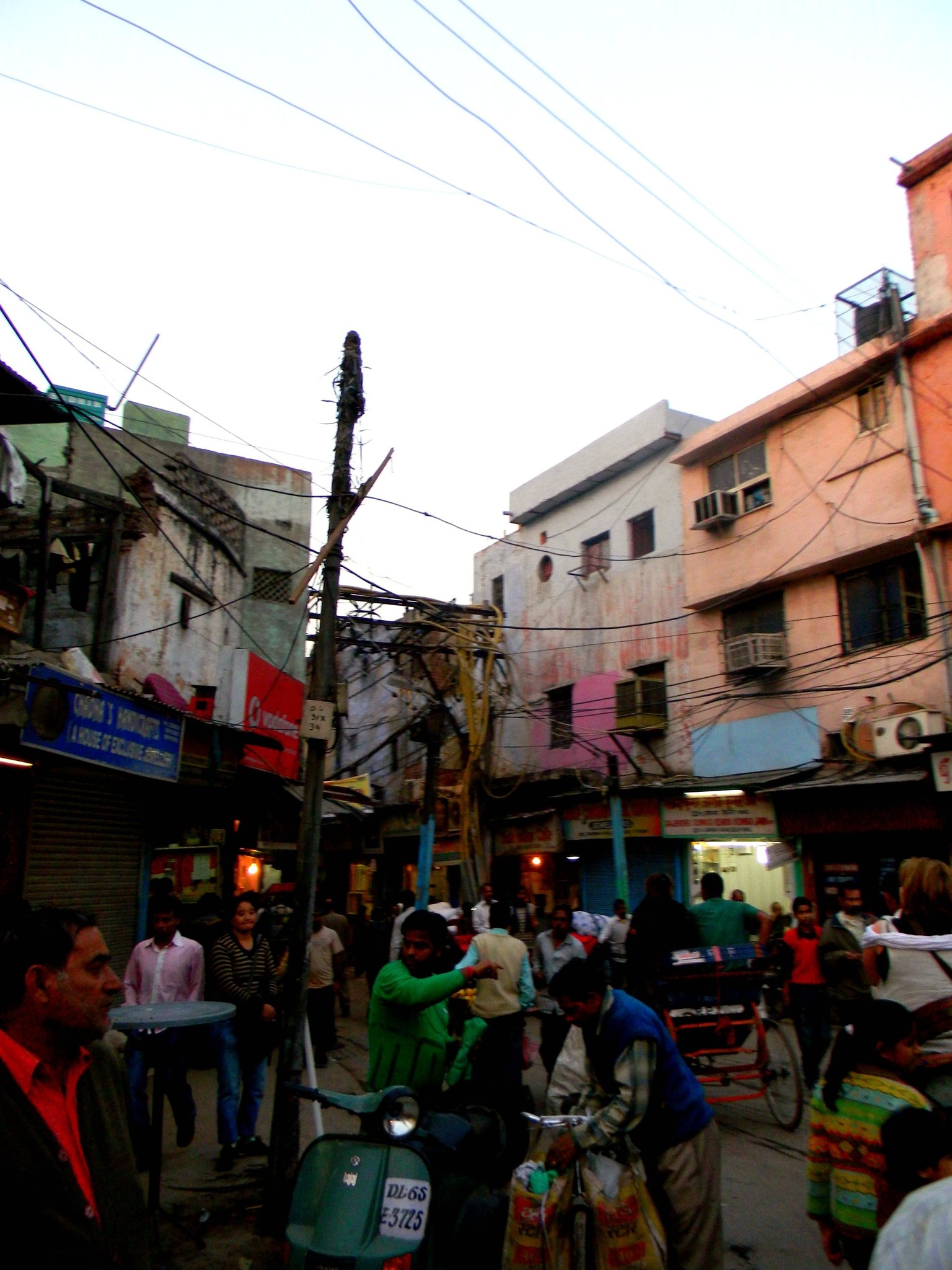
[106,1001,238,1249]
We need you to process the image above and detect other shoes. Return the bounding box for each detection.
[215,1147,240,1172]
[235,1137,270,1155]
[137,1146,153,1170]
[176,1120,195,1147]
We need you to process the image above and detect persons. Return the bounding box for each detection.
[867,1105,951,1269]
[541,957,724,1270]
[363,884,588,1147]
[209,897,278,1171]
[1,905,156,1270]
[253,886,370,1067]
[819,855,952,1063]
[775,897,832,1087]
[152,878,174,896]
[123,902,204,1169]
[598,872,773,1017]
[803,1001,932,1270]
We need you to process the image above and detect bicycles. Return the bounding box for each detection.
[507,1087,664,1270]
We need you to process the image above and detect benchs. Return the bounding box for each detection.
[656,943,767,1056]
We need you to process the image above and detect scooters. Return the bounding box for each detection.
[272,1079,504,1270]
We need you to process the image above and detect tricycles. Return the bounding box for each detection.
[642,941,813,1136]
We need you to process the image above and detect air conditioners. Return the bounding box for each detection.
[721,632,787,673]
[871,709,945,759]
[690,490,740,531]
[613,677,670,729]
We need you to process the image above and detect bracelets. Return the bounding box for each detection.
[466,967,476,978]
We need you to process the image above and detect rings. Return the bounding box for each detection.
[552,1161,555,1165]
